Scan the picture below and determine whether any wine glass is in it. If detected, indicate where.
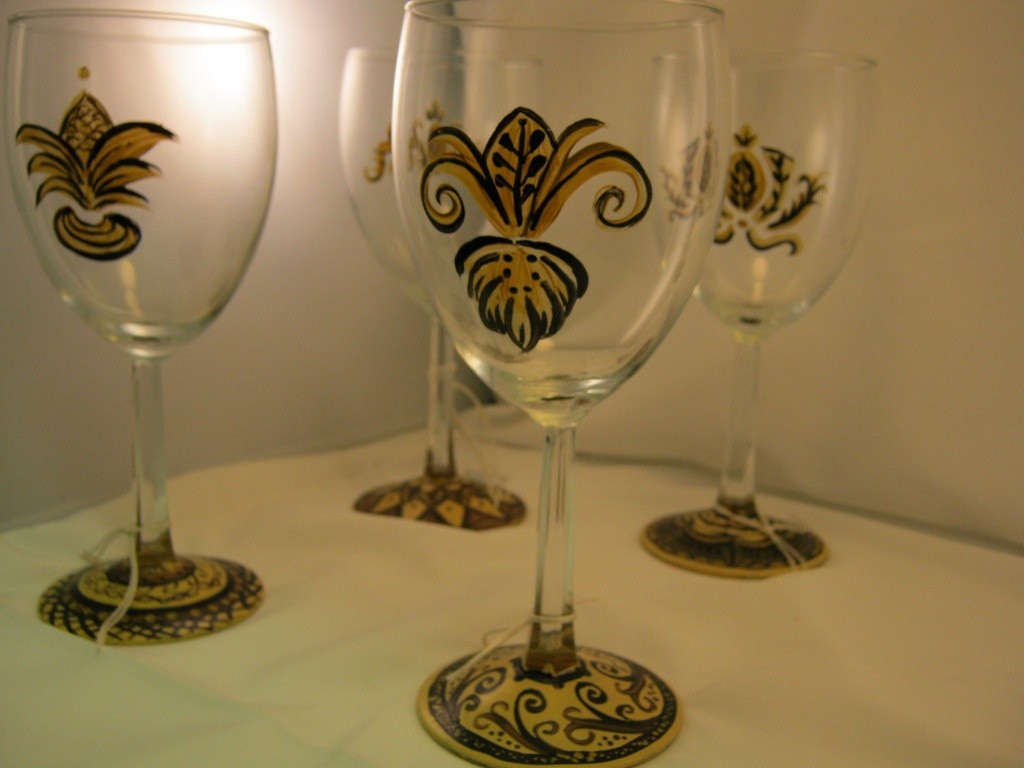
[640,44,883,579]
[337,45,526,534]
[385,0,728,768]
[0,7,280,644]
